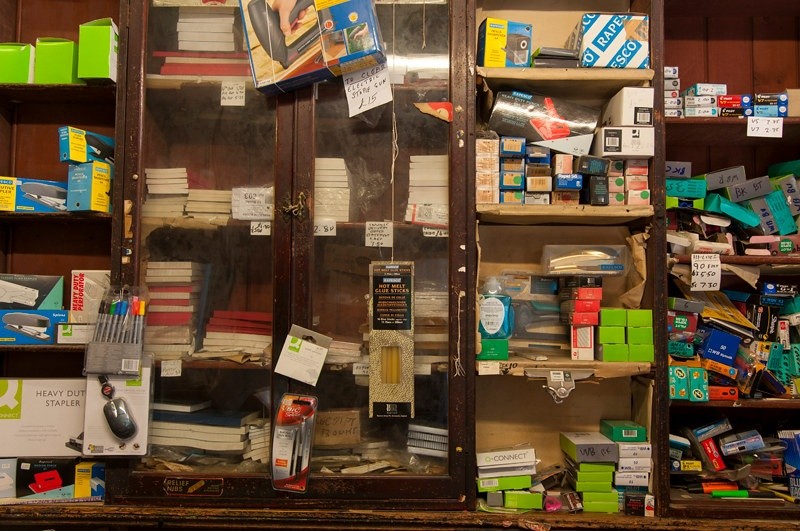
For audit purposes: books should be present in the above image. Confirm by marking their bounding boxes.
[146,399,270,466]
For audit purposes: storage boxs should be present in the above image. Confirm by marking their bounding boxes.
[476,419,654,516]
[478,242,654,363]
[563,11,650,69]
[0,269,112,345]
[239,2,387,95]
[0,16,119,85]
[664,161,798,261]
[0,374,107,508]
[662,285,800,407]
[475,86,654,205]
[667,421,799,507]
[477,17,534,69]
[1,124,113,217]
[664,67,799,121]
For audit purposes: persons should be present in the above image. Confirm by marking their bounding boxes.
[272,0,305,37]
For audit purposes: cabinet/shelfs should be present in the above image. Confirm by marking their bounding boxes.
[475,1,660,529]
[130,1,471,516]
[0,0,124,528]
[666,0,800,530]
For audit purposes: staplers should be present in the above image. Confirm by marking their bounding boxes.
[85,133,113,162]
[2,313,51,340]
[20,182,68,210]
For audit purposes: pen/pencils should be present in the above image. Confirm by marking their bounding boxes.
[94,296,144,343]
[296,425,309,472]
[712,489,776,498]
[759,485,800,503]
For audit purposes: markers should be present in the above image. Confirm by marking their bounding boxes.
[688,482,744,495]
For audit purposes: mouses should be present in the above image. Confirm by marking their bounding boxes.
[103,397,137,440]
